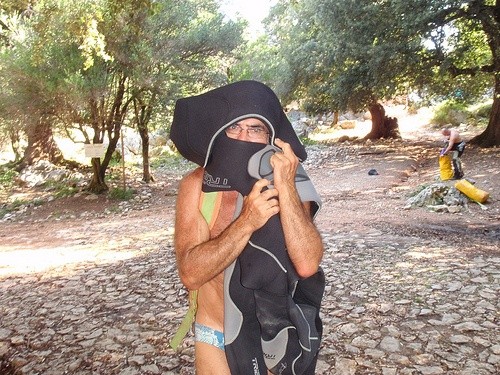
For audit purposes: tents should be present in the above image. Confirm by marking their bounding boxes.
[454,178,490,205]
[438,153,455,181]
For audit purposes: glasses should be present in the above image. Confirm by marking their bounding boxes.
[227,124,271,139]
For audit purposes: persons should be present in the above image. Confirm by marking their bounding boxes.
[169,78,325,375]
[440,127,467,181]
